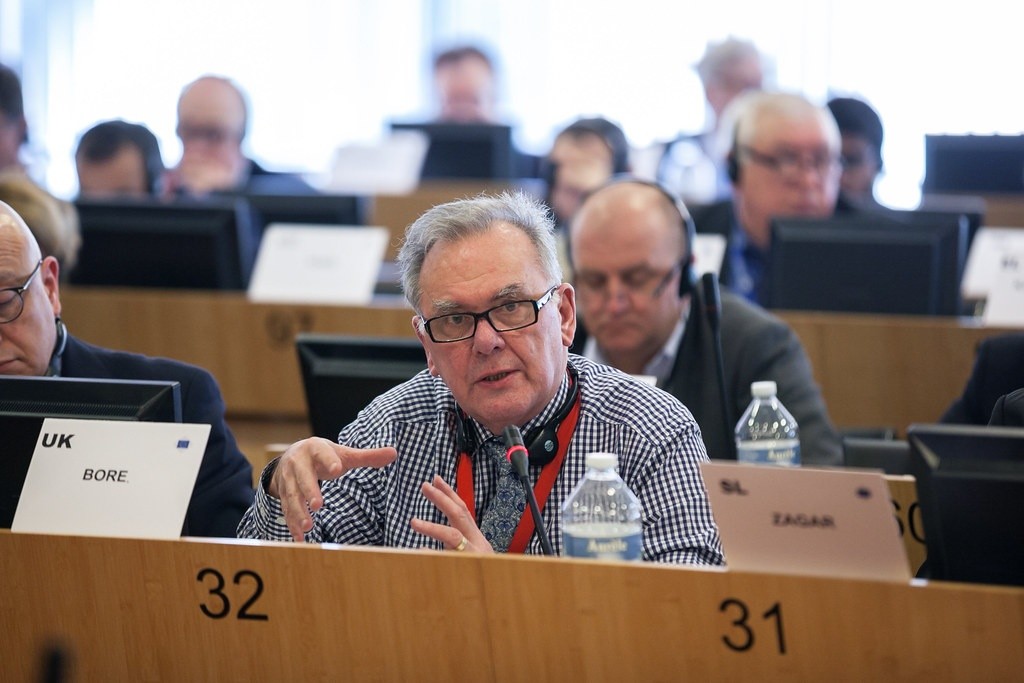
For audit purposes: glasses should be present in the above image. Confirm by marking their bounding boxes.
[735,143,840,175]
[419,284,559,343]
[0,260,43,324]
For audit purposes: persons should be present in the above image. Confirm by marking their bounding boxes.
[655,33,887,307]
[0,198,252,537]
[233,189,724,568]
[171,75,318,287]
[75,119,179,206]
[0,61,81,278]
[937,336,1024,428]
[430,44,543,179]
[558,182,844,469]
[547,117,631,287]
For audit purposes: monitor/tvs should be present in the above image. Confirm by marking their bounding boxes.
[0,118,1024,592]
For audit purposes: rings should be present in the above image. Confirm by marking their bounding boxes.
[455,535,469,550]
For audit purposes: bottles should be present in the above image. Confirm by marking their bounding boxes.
[558,448,645,562]
[734,380,802,468]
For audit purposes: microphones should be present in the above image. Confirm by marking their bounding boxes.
[503,424,554,555]
[702,271,736,466]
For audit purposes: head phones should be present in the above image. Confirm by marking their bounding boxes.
[723,124,745,184]
[567,177,698,297]
[455,360,579,464]
[45,322,67,380]
